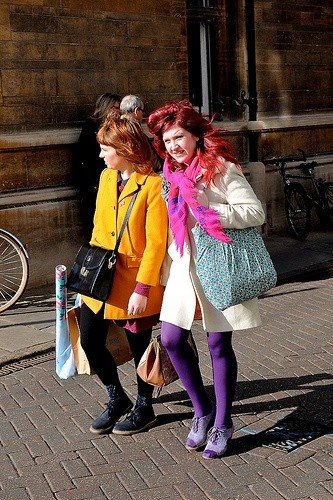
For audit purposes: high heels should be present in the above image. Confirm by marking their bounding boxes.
[201,418,235,459]
[186,405,216,450]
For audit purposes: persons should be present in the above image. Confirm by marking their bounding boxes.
[75,109,169,436]
[77,92,144,242]
[147,99,266,460]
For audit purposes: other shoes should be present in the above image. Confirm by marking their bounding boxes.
[89,393,135,434]
[111,404,158,434]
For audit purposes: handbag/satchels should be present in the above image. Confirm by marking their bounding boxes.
[66,241,119,301]
[74,151,94,181]
[191,220,278,310]
[64,304,135,375]
[137,329,200,388]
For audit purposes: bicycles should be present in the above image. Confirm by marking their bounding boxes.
[263,148,333,240]
[0,229,30,312]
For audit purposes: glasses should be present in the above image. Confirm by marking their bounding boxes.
[133,105,147,115]
